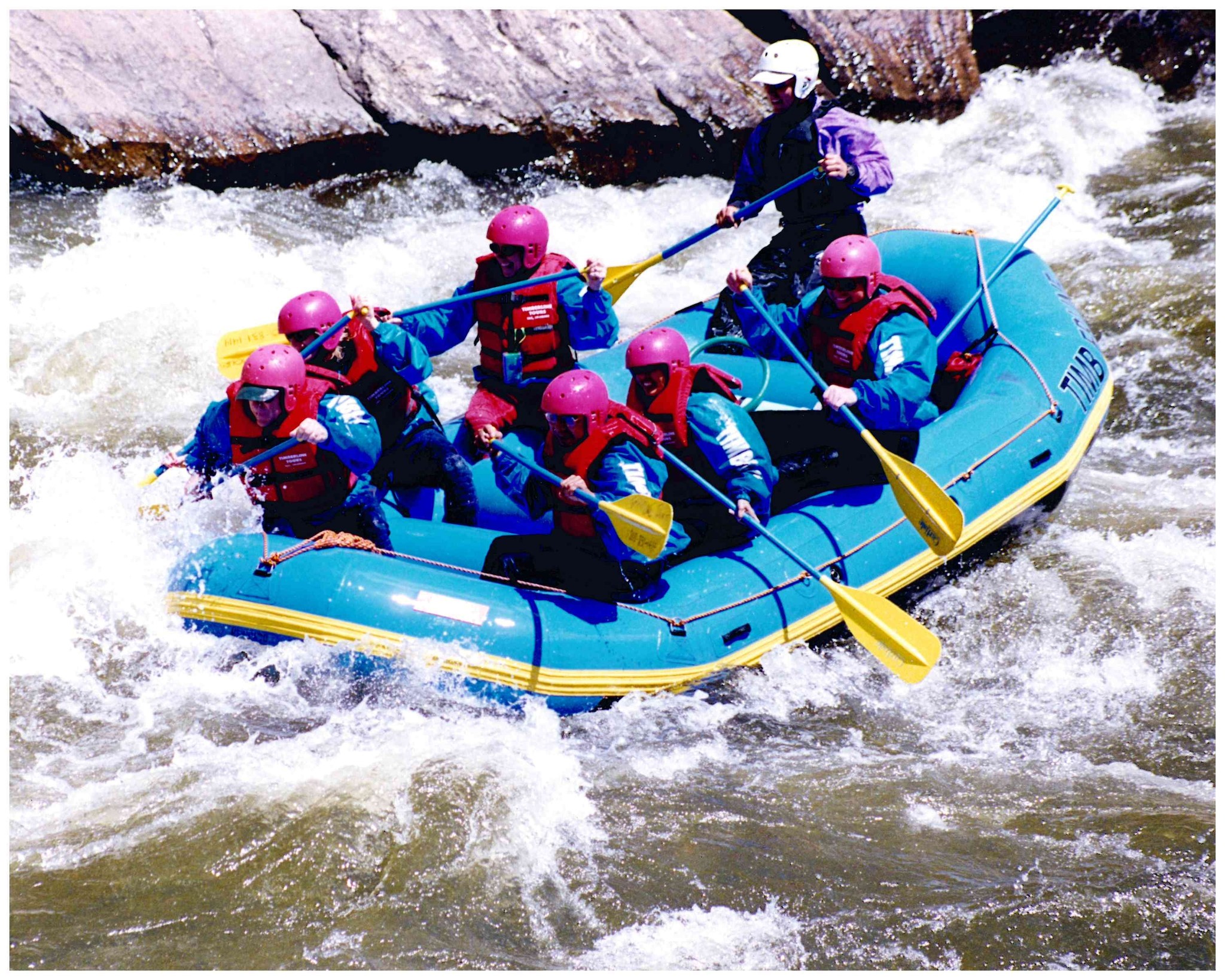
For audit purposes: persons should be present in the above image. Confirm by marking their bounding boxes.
[162,292,479,527]
[473,370,669,602]
[727,235,938,518]
[182,343,397,560]
[702,39,894,358]
[625,326,775,571]
[380,204,620,467]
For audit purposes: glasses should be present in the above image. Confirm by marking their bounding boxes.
[545,412,585,428]
[490,243,523,257]
[763,81,786,90]
[822,276,860,292]
[285,328,317,342]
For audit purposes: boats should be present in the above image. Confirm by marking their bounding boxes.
[166,227,1112,723]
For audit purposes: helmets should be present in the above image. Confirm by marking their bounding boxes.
[277,291,345,350]
[486,205,549,268]
[750,39,822,99]
[233,344,306,424]
[625,328,689,380]
[820,235,880,299]
[541,370,610,431]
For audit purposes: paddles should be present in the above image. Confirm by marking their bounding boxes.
[486,436,674,560]
[656,442,942,687]
[739,279,969,559]
[933,184,1075,350]
[132,435,305,524]
[138,307,369,489]
[580,164,825,307]
[219,264,590,380]
